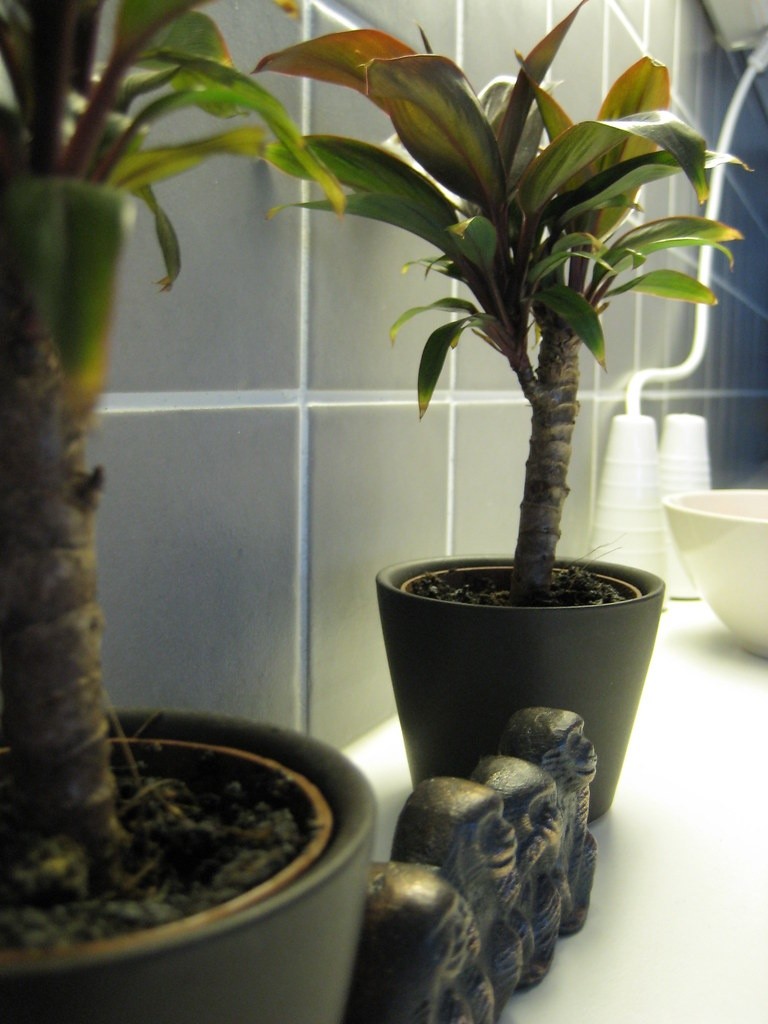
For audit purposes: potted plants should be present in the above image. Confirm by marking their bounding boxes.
[1,1,391,1023]
[249,0,758,823]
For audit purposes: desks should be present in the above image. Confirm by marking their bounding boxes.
[337,582,768,1024]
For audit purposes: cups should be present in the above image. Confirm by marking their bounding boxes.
[588,411,713,611]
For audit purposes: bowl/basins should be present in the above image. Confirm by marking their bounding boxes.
[660,488,768,662]
